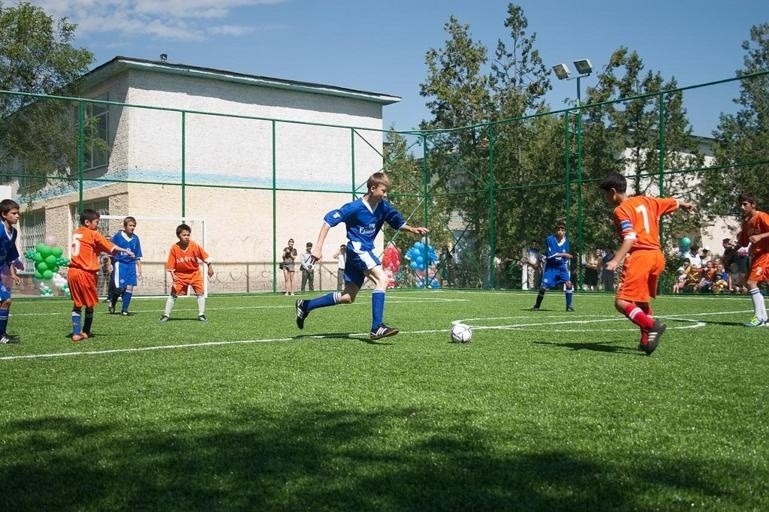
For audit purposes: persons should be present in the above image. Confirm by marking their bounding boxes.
[599,248,618,293]
[278,239,298,296]
[598,172,695,355]
[529,222,576,312]
[158,224,215,323]
[435,246,457,287]
[491,248,506,292]
[583,248,603,293]
[0,199,23,345]
[727,192,769,328]
[332,243,347,292]
[295,171,432,340]
[299,242,316,293]
[523,240,544,290]
[668,238,752,295]
[107,216,143,317]
[99,235,112,298]
[67,208,135,343]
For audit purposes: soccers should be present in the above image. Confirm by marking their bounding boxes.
[451,323,472,342]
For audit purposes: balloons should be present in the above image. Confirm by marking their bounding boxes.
[382,241,441,288]
[24,242,70,296]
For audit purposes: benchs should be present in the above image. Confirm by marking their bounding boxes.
[687,282,696,293]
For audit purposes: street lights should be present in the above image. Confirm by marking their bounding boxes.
[549,59,589,291]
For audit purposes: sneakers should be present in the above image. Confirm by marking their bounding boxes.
[0,334,20,344]
[72,331,94,341]
[745,315,765,327]
[295,299,311,329]
[160,313,168,321]
[122,311,133,315]
[109,301,115,313]
[646,320,666,355]
[370,323,398,341]
[198,315,208,322]
